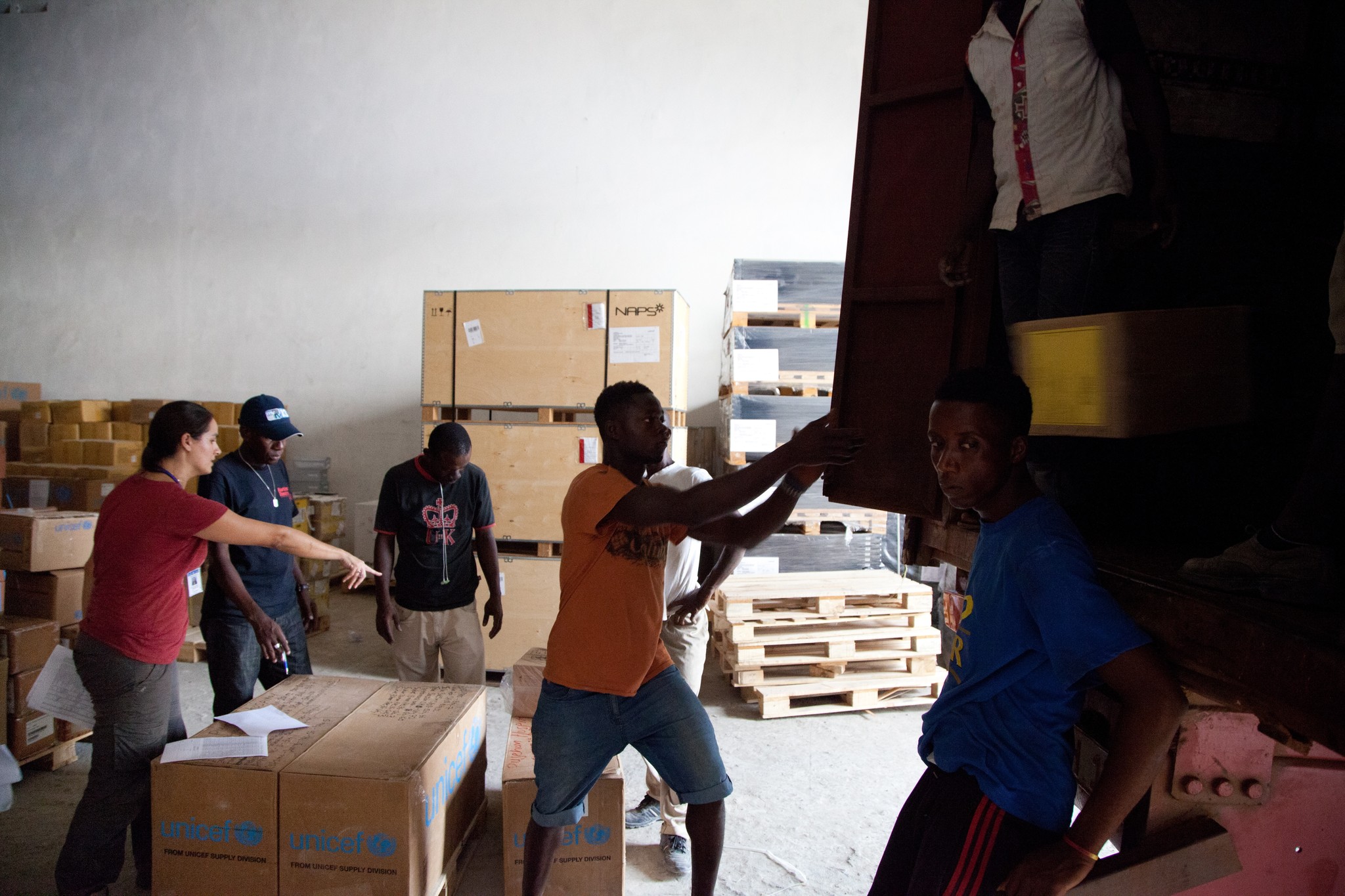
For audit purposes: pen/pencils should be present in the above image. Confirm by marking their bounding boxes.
[283,650,291,676]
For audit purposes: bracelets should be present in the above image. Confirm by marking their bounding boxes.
[295,581,311,594]
[1059,835,1103,863]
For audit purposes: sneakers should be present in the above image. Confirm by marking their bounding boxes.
[625,794,663,829]
[658,833,692,874]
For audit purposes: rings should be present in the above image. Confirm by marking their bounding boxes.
[273,642,281,649]
[358,570,362,574]
[673,612,680,618]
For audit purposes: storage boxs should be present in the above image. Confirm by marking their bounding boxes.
[151,674,391,894]
[512,646,548,719]
[419,420,603,544]
[278,681,492,896]
[419,290,674,411]
[0,382,344,768]
[501,714,626,896]
[1009,307,1216,438]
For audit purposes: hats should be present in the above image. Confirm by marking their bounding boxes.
[238,394,304,442]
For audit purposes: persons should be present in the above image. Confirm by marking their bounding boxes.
[845,353,1184,896]
[50,403,389,896]
[949,1,1147,353]
[511,379,839,896]
[372,423,505,684]
[617,405,746,880]
[196,391,321,727]
